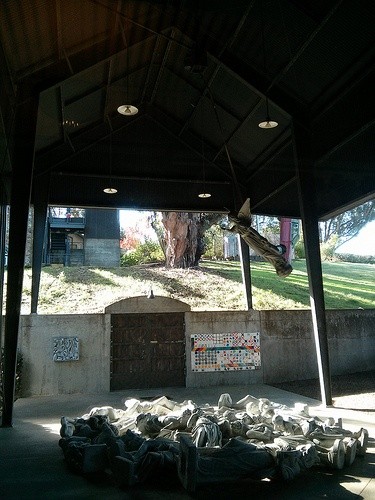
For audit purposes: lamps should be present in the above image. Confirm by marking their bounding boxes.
[197,140,211,198]
[117,69,138,116]
[103,136,117,194]
[258,94,279,129]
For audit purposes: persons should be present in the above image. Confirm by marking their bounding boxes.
[272,415,363,442]
[176,433,320,490]
[58,414,147,480]
[91,394,195,419]
[191,415,223,449]
[218,394,309,426]
[137,408,203,438]
[112,438,176,490]
[232,420,357,465]
[220,197,293,279]
